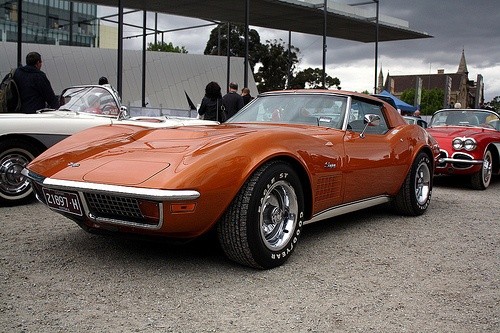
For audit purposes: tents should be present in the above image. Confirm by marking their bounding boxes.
[377,89,419,113]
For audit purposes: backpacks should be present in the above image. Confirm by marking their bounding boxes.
[0,68,22,113]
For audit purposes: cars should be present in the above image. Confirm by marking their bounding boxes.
[425,109,500,189]
[403,117,428,128]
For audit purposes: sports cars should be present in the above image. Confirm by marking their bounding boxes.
[0,86,221,205]
[21,90,440,267]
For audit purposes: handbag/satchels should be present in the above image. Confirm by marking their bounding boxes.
[220,111,228,123]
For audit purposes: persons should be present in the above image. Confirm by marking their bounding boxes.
[241,87,255,106]
[98,76,122,107]
[198,81,223,123]
[84,94,103,114]
[223,82,244,122]
[0,52,60,114]
[446,102,461,127]
